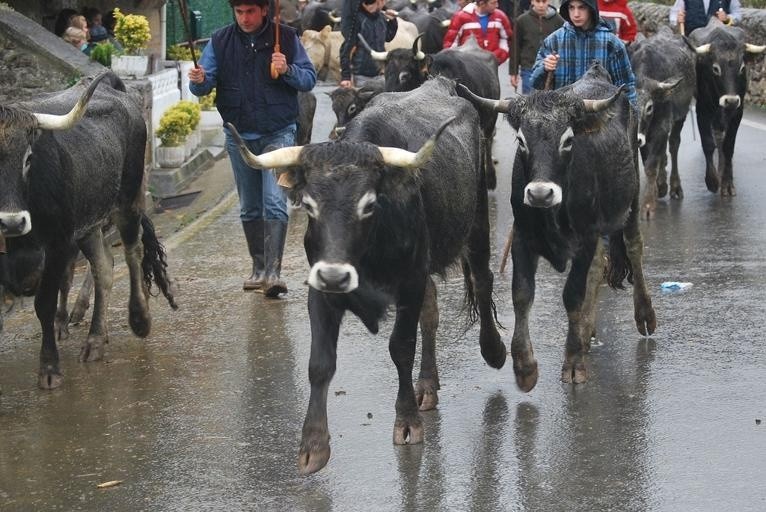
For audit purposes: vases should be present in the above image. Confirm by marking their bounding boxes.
[107,5,151,79]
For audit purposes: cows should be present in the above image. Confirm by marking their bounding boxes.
[458,59,656,392]
[0,217,117,340]
[357,32,501,190]
[323,81,387,139]
[625,26,697,221]
[224,72,507,477]
[679,15,766,197]
[0,71,178,390]
[278,0,459,86]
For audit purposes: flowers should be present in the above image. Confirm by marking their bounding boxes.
[151,86,221,167]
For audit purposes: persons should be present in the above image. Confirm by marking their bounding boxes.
[56,8,124,65]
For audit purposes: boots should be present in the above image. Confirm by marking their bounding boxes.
[242,220,264,290]
[262,218,289,297]
